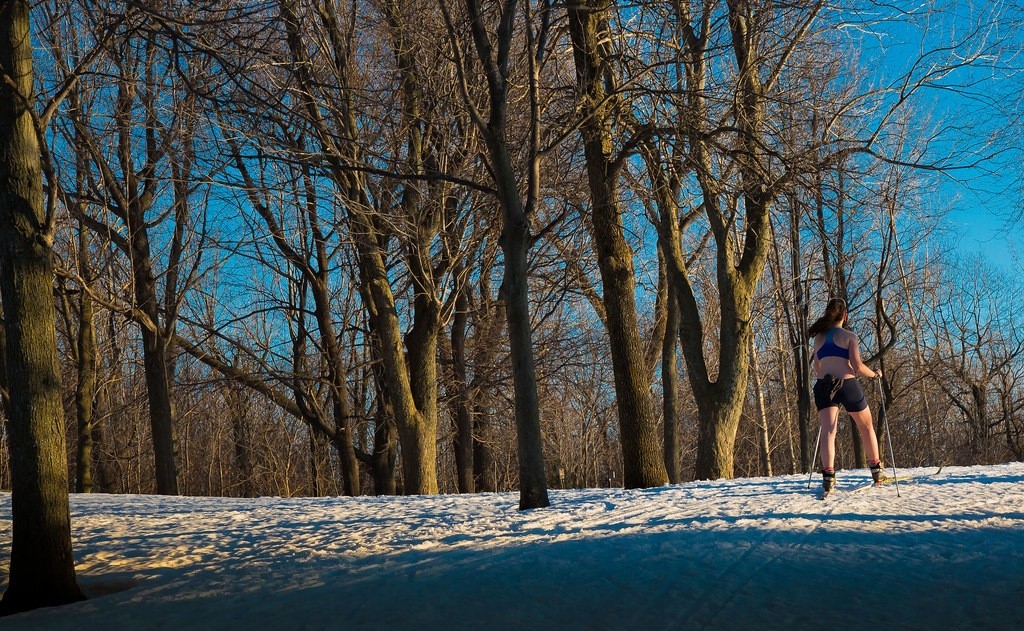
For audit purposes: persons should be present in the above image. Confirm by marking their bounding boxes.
[805,300,883,498]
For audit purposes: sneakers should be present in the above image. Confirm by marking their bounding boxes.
[820,469,836,491]
[866,460,887,483]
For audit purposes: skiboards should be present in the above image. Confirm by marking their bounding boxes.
[819,465,942,501]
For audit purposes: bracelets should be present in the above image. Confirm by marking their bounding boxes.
[873,373,879,379]
